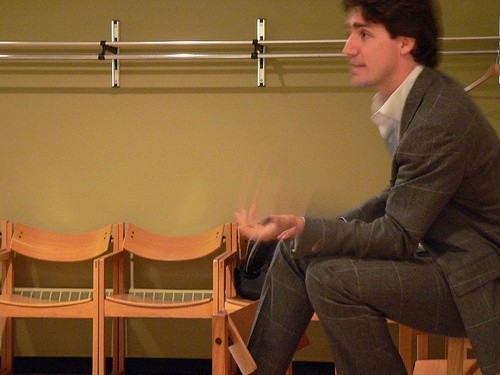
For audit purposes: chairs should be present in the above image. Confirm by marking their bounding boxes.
[0,218,498,375]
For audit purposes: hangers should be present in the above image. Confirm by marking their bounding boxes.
[463,53,499,94]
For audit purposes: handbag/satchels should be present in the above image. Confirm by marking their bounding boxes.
[234,223,276,300]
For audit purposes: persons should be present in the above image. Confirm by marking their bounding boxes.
[234,0,500,375]
